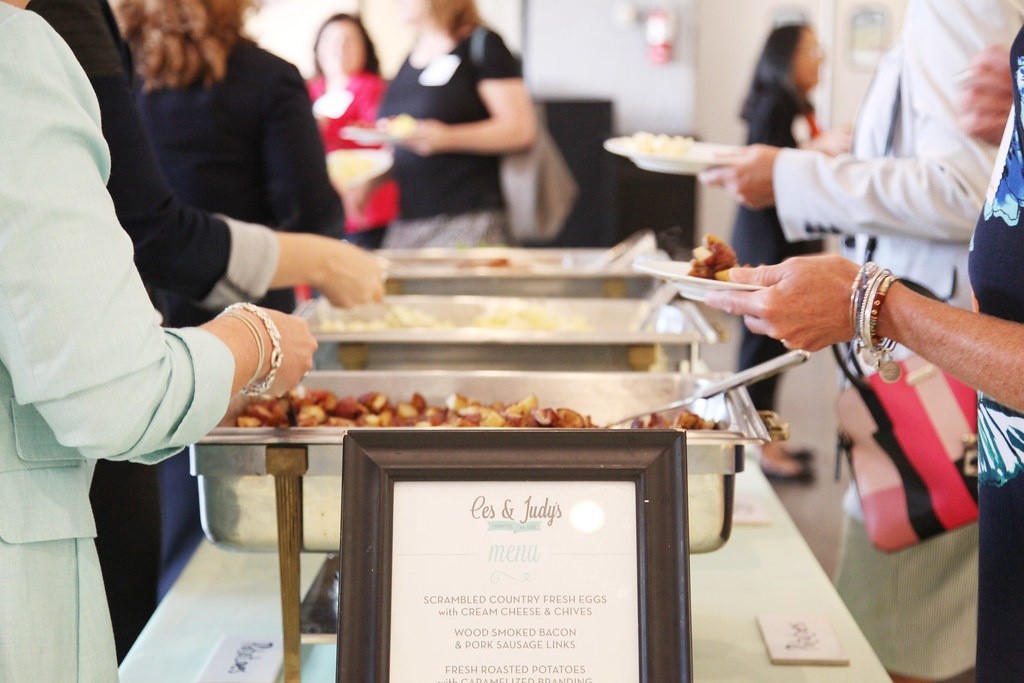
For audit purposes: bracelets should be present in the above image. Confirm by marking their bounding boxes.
[223,302,284,396]
[849,261,903,381]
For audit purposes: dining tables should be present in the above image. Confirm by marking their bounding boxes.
[109,365,895,680]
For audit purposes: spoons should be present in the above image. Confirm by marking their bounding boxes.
[591,229,657,274]
[630,280,680,332]
[601,346,808,431]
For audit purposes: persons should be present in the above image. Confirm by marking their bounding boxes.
[706,21,1024,683]
[727,23,828,484]
[623,10,699,138]
[0,0,318,683]
[379,0,542,247]
[105,0,347,612]
[303,15,396,252]
[698,2,1024,682]
[0,2,388,662]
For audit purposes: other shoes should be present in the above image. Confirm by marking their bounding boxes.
[761,450,816,488]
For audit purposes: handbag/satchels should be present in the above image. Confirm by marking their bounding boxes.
[838,347,982,554]
[496,105,578,238]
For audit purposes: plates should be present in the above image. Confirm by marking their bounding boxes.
[339,125,424,146]
[327,150,395,184]
[631,258,771,302]
[603,136,745,176]
